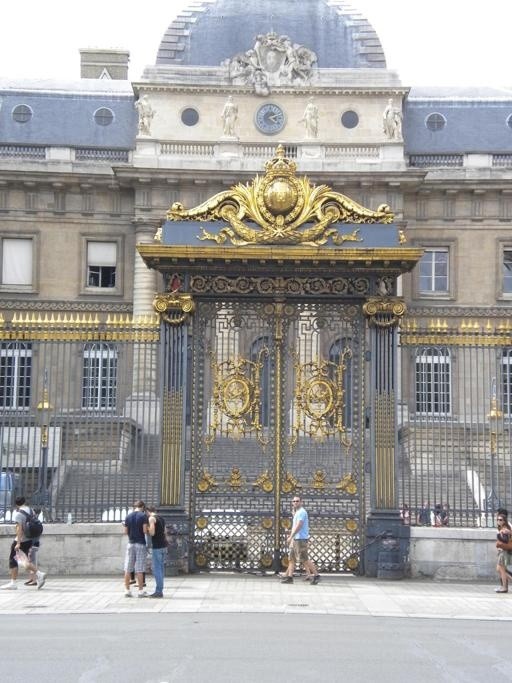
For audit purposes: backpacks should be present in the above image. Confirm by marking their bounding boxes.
[16,506,43,538]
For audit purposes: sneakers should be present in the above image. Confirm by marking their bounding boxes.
[124,590,163,598]
[0,581,19,590]
[303,573,320,586]
[24,570,47,590]
[277,571,294,584]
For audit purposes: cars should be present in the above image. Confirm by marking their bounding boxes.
[100,505,140,522]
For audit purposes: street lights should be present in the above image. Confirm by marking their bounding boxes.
[37,386,55,520]
[485,392,504,514]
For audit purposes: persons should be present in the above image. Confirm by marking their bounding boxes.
[122,499,148,597]
[0,495,48,590]
[282,496,321,585]
[302,95,318,139]
[139,94,155,135]
[383,98,402,139]
[23,506,40,586]
[125,506,151,587]
[399,501,451,527]
[146,504,169,597]
[280,504,314,581]
[222,94,237,134]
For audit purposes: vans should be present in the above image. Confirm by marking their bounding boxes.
[195,508,252,562]
[1,472,25,521]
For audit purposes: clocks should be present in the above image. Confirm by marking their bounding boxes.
[255,101,287,133]
